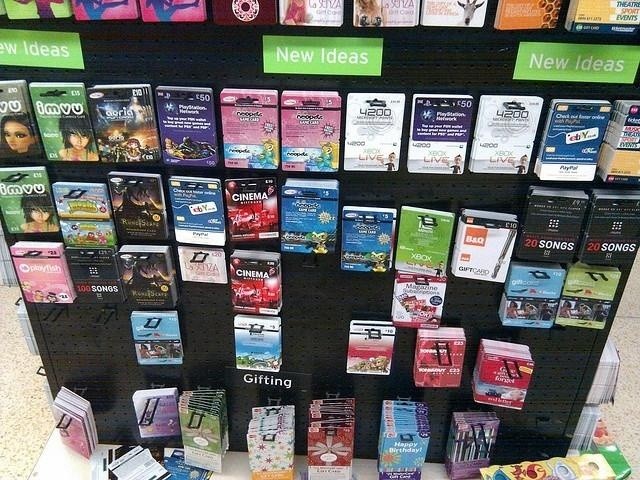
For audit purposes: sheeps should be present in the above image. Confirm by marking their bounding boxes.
[457,0,485,26]
